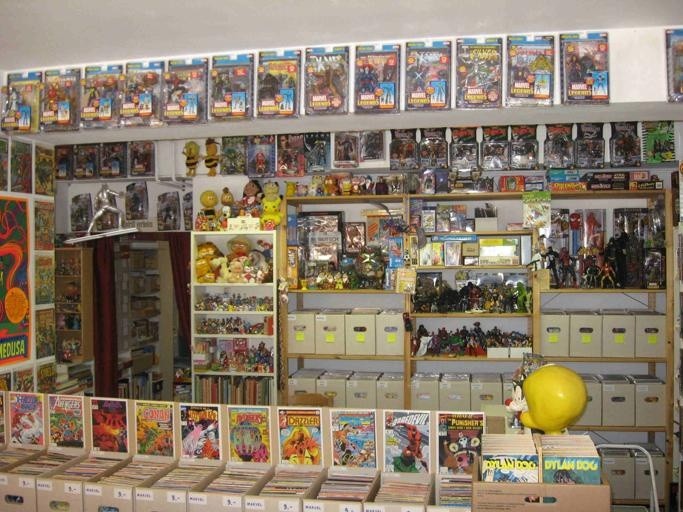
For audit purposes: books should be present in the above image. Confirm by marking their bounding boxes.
[109,372,272,406]
[1,391,610,510]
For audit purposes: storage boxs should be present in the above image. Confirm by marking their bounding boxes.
[345,371,382,407]
[289,309,323,354]
[511,347,532,358]
[488,347,510,358]
[0,99,683,512]
[316,369,352,408]
[409,371,440,410]
[630,311,664,357]
[376,372,404,408]
[498,369,515,406]
[630,441,663,501]
[344,308,381,356]
[566,374,601,427]
[565,311,603,358]
[598,311,636,359]
[543,312,569,356]
[314,310,349,355]
[597,374,635,427]
[470,372,503,413]
[598,445,636,501]
[288,367,324,395]
[376,309,404,356]
[628,372,665,427]
[438,371,471,411]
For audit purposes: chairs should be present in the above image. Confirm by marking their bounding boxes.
[283,390,336,408]
